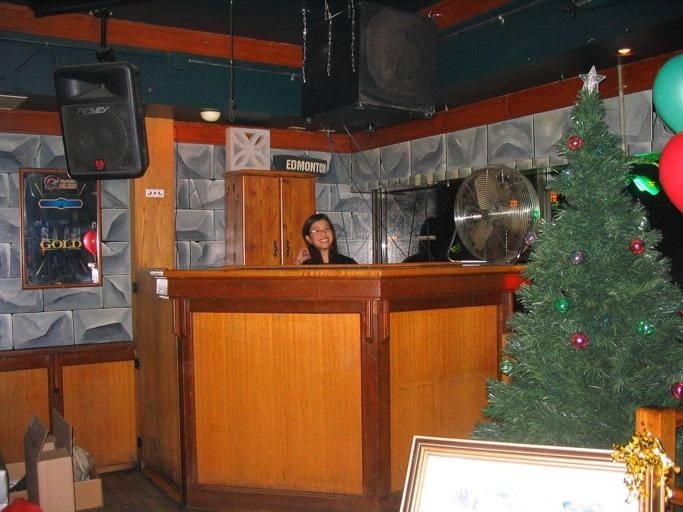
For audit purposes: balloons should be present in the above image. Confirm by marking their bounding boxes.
[658,131,683,213]
[652,54,683,134]
[82,231,97,257]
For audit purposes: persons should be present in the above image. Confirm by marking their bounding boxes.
[300,213,359,265]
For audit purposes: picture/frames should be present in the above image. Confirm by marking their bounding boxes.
[398,434,667,512]
[17,165,104,291]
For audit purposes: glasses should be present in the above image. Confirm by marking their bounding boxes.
[306,226,333,236]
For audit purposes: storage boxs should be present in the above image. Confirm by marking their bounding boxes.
[4,408,106,511]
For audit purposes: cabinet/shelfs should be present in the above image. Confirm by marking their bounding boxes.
[222,169,317,269]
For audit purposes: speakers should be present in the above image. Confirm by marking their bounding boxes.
[53,62,150,181]
[300,1,439,134]
